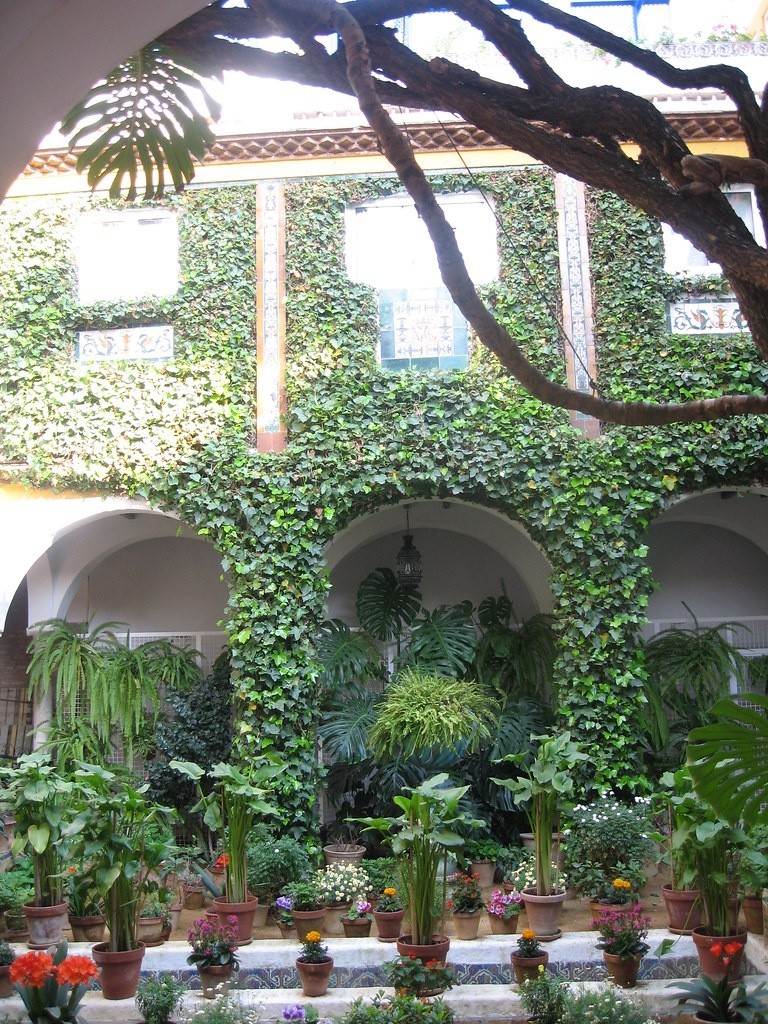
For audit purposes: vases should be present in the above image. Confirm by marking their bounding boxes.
[366,896,382,913]
[488,913,519,934]
[520,833,567,871]
[340,912,372,937]
[373,907,405,937]
[452,908,484,940]
[296,955,334,997]
[277,920,298,939]
[199,962,234,998]
[252,904,271,927]
[510,949,548,988]
[589,900,633,928]
[693,1010,746,1024]
[603,950,641,988]
[211,867,227,892]
[321,901,353,934]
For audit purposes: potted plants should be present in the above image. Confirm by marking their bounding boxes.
[489,731,592,935]
[280,881,327,943]
[465,838,503,887]
[168,760,281,940]
[644,792,703,928]
[342,772,487,987]
[323,812,367,868]
[497,843,536,910]
[0,751,207,1000]
[659,757,747,980]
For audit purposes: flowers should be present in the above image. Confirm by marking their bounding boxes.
[216,850,232,868]
[485,890,522,919]
[509,964,662,1024]
[598,879,633,907]
[664,940,768,1024]
[590,904,655,961]
[338,950,462,1024]
[517,927,541,958]
[134,970,270,1024]
[186,912,242,970]
[275,857,400,916]
[297,929,331,964]
[512,856,568,894]
[560,791,653,839]
[8,936,102,1024]
[274,1002,336,1024]
[446,871,486,913]
